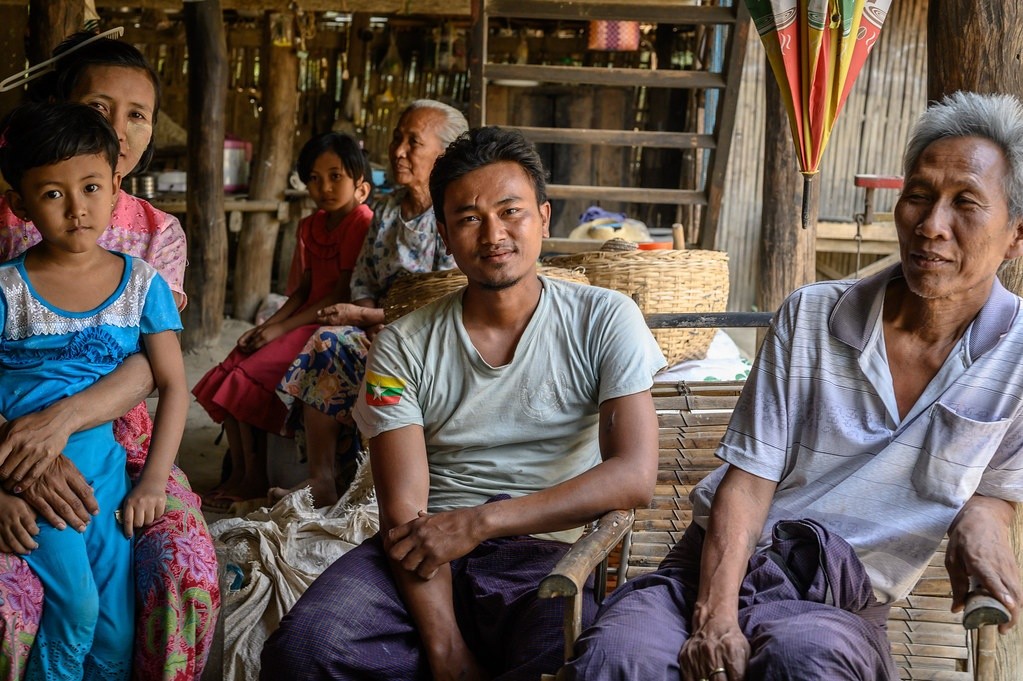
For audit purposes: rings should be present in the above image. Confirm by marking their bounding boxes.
[707,667,726,677]
[699,678,710,681]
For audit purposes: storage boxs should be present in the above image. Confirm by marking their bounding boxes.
[223,140,252,192]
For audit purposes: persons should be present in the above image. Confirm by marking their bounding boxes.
[225,100,469,508]
[353,157,374,209]
[198,130,377,512]
[1,96,190,681]
[259,121,658,681]
[0,33,225,681]
[553,88,1022,681]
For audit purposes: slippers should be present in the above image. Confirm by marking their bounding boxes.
[201,476,277,511]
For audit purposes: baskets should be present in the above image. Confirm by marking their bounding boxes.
[541,249,729,373]
[383,264,589,323]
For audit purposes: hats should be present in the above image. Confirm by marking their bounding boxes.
[567,217,654,242]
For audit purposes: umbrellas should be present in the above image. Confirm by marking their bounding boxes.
[745,0,893,230]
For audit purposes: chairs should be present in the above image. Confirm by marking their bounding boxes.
[534,307,1023,681]
